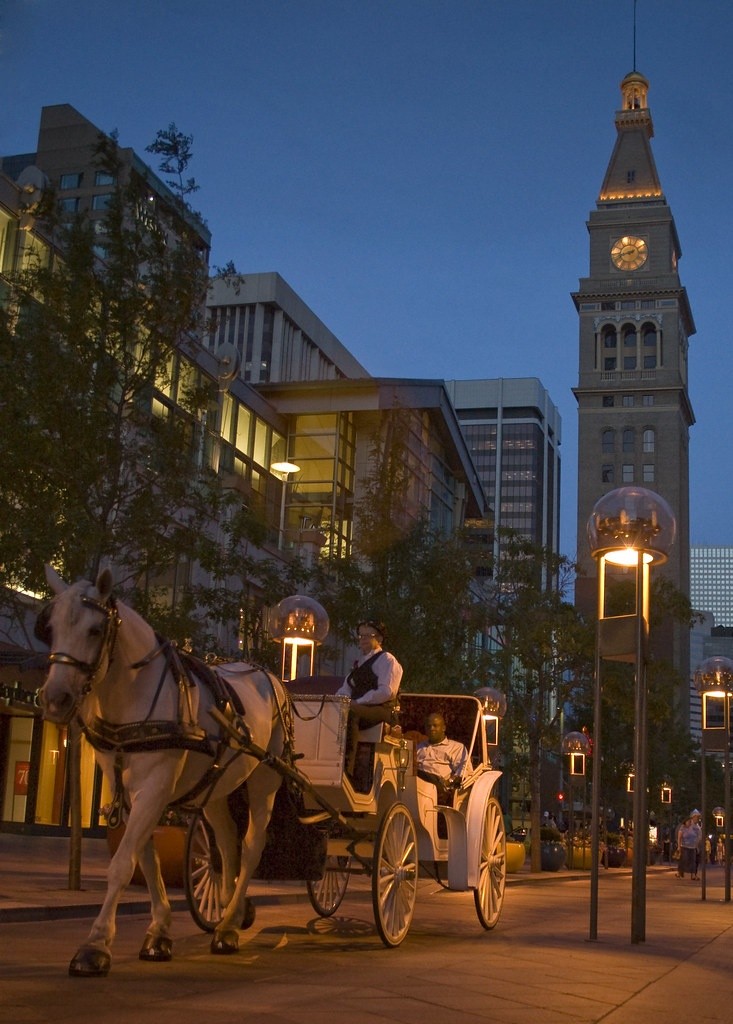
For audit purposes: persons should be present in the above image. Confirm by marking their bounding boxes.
[501,808,513,836]
[677,808,703,880]
[616,826,634,837]
[600,821,604,837]
[416,712,473,836]
[706,836,725,866]
[662,835,670,864]
[543,812,568,836]
[335,617,404,791]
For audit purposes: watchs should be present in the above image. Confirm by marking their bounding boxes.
[449,778,454,783]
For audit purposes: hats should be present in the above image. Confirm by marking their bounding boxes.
[356,618,386,637]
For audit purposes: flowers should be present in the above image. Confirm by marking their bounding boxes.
[561,829,607,852]
[97,795,203,827]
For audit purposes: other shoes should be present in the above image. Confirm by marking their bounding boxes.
[691,875,700,880]
[675,873,682,880]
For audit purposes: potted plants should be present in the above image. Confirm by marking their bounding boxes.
[598,832,625,868]
[537,826,562,868]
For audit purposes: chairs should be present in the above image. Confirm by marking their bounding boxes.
[283,675,394,796]
[398,693,491,839]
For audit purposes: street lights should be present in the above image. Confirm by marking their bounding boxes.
[267,594,329,683]
[694,657,733,903]
[712,807,724,835]
[623,759,633,871]
[657,774,676,864]
[558,732,588,871]
[474,687,506,768]
[584,486,676,950]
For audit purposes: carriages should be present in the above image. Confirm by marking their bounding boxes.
[34,554,508,979]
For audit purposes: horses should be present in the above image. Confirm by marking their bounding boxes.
[38,562,295,979]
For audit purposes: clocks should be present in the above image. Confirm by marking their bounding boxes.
[611,236,648,271]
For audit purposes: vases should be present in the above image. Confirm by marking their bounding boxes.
[562,845,602,870]
[506,838,524,872]
[106,824,185,890]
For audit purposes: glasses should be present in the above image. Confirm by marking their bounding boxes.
[359,633,376,639]
